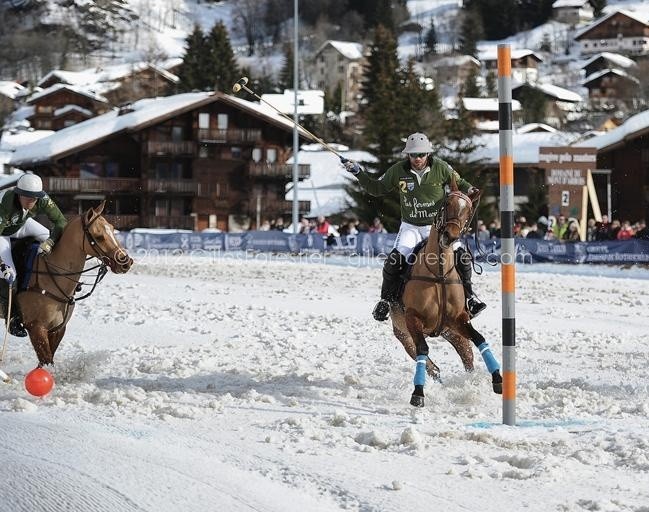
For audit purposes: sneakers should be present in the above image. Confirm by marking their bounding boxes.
[469,298,486,315]
[374,300,392,317]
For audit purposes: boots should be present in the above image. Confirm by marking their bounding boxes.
[2,303,27,337]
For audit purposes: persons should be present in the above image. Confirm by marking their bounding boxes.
[259,215,387,234]
[0,172,68,337]
[340,131,487,321]
[461,213,649,241]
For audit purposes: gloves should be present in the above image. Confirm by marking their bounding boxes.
[1,263,15,282]
[468,187,480,198]
[37,238,54,255]
[341,158,360,175]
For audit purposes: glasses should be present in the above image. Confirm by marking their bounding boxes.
[409,153,427,158]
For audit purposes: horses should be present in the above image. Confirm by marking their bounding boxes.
[0,200,133,368]
[389,171,503,408]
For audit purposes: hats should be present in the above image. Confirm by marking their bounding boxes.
[12,172,46,198]
[402,132,436,154]
